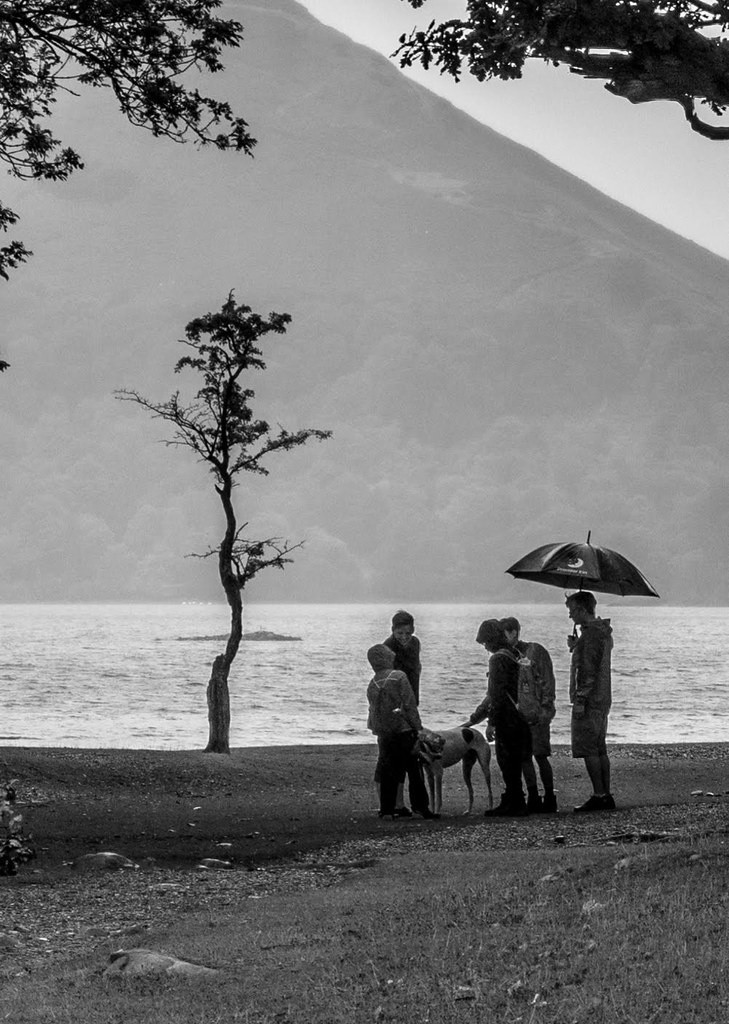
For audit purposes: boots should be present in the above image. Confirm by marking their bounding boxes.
[485,792,526,816]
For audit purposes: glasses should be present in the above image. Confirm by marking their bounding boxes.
[569,608,577,615]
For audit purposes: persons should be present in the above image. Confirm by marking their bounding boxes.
[367,610,439,818]
[457,613,557,817]
[565,591,615,812]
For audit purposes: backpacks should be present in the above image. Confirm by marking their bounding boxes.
[370,670,405,737]
[494,646,543,726]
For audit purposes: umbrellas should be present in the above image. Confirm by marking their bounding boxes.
[504,530,660,652]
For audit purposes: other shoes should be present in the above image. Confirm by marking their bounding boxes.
[411,806,440,819]
[542,795,557,812]
[395,808,412,817]
[527,796,544,812]
[573,794,616,812]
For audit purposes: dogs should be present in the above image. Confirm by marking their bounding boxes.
[417,725,494,815]
